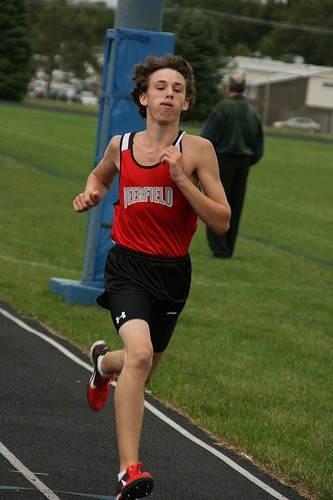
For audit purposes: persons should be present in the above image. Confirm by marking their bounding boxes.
[72,55,231,500]
[200,75,264,259]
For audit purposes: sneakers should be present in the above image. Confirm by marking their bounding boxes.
[87,340,115,412]
[115,461,154,500]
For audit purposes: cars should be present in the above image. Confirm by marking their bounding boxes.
[73,90,97,105]
[273,116,321,132]
